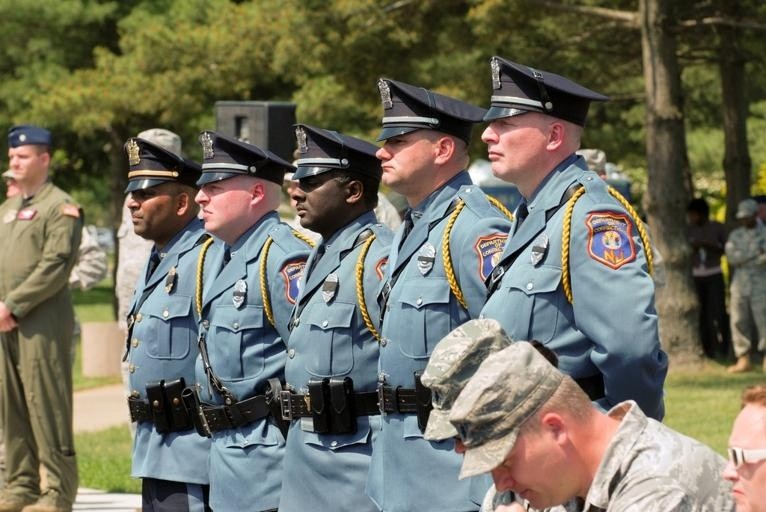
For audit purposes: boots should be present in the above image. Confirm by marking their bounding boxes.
[728,353,753,373]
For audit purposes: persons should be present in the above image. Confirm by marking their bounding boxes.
[446,337,737,512]
[282,159,405,247]
[718,382,766,512]
[575,149,607,182]
[271,117,398,511]
[62,224,109,294]
[477,52,670,426]
[0,122,87,512]
[121,133,229,512]
[684,196,732,359]
[753,194,766,227]
[724,198,766,376]
[373,75,515,512]
[3,168,21,199]
[191,128,321,512]
[112,127,185,439]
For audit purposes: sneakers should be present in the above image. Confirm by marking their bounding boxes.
[22,491,71,512]
[0,492,26,512]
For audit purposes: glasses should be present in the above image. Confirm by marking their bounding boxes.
[724,445,766,473]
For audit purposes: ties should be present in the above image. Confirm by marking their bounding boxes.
[307,243,325,280]
[221,248,230,273]
[144,251,158,284]
[396,207,414,250]
[514,201,528,234]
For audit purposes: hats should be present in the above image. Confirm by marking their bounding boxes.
[483,55,611,128]
[7,125,53,148]
[123,136,200,193]
[290,121,383,182]
[447,340,566,483]
[137,125,183,158]
[736,200,759,220]
[195,129,298,186]
[576,148,606,173]
[374,76,489,143]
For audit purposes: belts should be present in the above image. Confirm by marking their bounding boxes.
[566,375,607,402]
[193,393,270,439]
[126,396,196,424]
[281,390,382,419]
[376,387,419,417]
